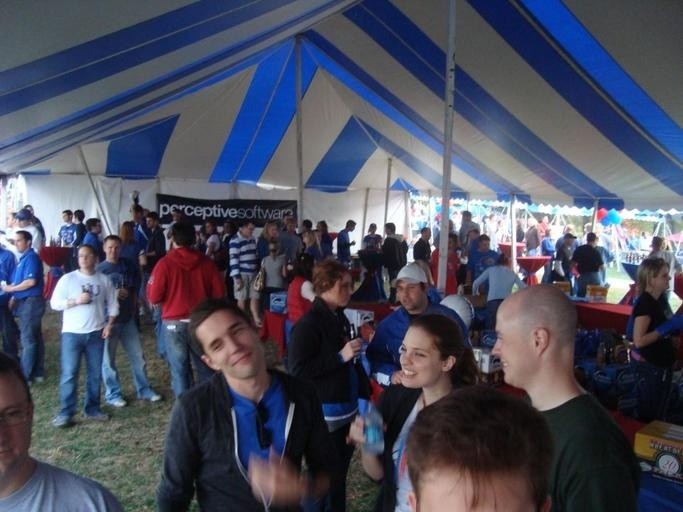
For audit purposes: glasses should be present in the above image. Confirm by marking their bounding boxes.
[256,403,272,449]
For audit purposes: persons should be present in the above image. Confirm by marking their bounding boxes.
[156,253,681,510]
[0,351,123,511]
[0,205,46,386]
[51,202,357,429]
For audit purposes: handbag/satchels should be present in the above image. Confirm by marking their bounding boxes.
[253,269,265,292]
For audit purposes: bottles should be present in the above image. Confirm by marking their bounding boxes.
[361,401,385,456]
[347,324,361,364]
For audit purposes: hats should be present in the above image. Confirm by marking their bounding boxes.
[13,209,32,219]
[390,263,427,287]
[564,234,577,239]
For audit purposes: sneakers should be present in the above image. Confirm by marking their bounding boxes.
[52,392,162,426]
